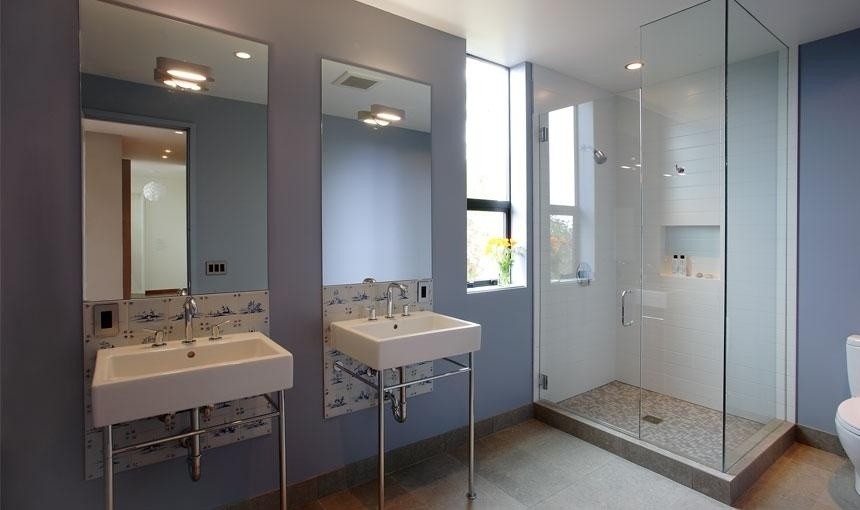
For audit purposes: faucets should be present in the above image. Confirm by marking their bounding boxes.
[363,278,376,285]
[386,281,408,318]
[183,298,197,342]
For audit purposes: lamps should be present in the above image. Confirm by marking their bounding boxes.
[358,104,406,127]
[153,56,212,91]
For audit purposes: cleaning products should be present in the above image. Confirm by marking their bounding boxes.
[672,254,677,276]
[678,255,686,276]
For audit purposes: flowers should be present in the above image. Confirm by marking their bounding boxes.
[483,234,525,285]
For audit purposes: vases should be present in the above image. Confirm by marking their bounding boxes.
[498,264,510,287]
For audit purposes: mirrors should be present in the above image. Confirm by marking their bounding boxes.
[320,56,432,286]
[79,0,268,303]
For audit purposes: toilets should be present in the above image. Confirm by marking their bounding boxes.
[836,334,860,488]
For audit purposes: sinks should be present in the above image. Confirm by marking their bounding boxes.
[634,286,684,310]
[91,330,294,427]
[330,310,482,371]
